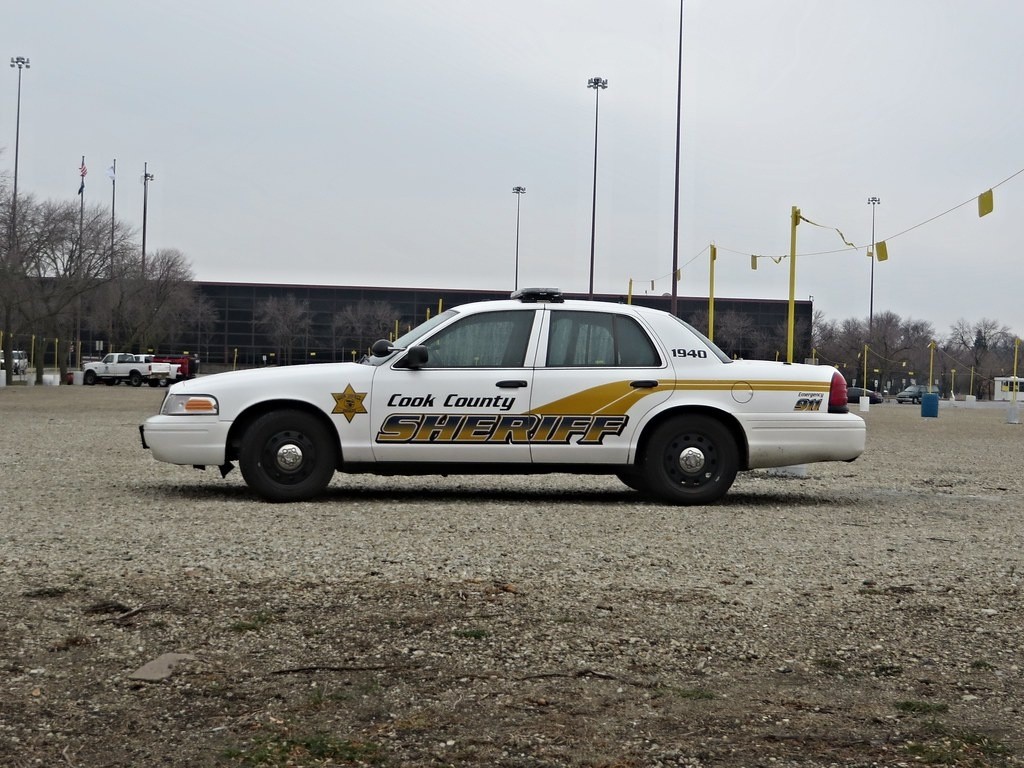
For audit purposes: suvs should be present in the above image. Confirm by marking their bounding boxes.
[0,348,28,376]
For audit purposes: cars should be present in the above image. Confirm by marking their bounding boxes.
[896,385,941,404]
[844,387,884,405]
[139,285,867,506]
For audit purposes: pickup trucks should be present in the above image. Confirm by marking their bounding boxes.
[112,354,185,386]
[82,352,171,387]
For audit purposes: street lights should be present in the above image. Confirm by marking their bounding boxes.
[587,77,608,302]
[864,196,881,388]
[5,56,31,384]
[513,186,527,292]
